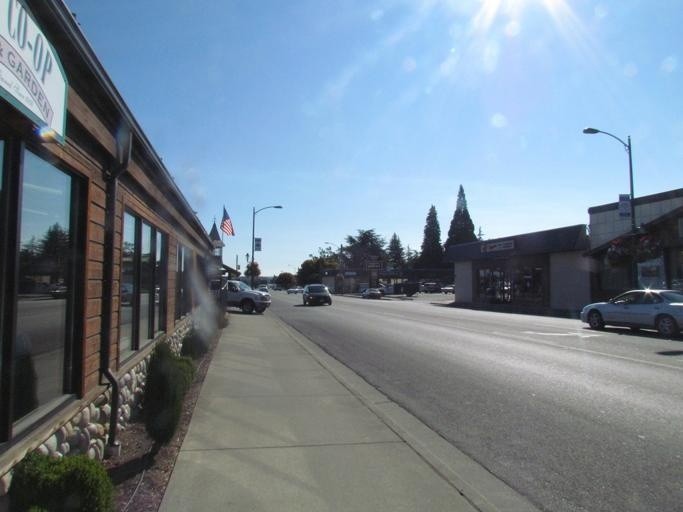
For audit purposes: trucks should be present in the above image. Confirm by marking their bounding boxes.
[376,272,419,296]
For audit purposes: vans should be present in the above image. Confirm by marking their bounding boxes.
[424,283,440,293]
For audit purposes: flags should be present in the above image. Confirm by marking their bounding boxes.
[219,208,235,236]
[208,223,225,248]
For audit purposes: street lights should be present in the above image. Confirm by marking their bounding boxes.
[583,128,639,289]
[251,205,282,290]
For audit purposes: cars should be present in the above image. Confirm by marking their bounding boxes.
[154,287,160,304]
[228,280,272,314]
[258,284,268,292]
[121,282,133,307]
[287,284,332,305]
[51,286,67,299]
[440,285,455,294]
[361,288,384,299]
[580,289,683,339]
